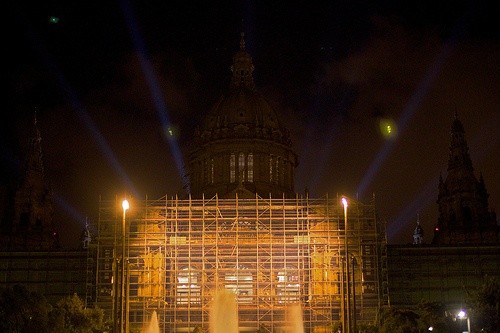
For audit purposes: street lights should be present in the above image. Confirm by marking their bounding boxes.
[457,311,471,333]
[341,197,352,333]
[120,200,130,333]
